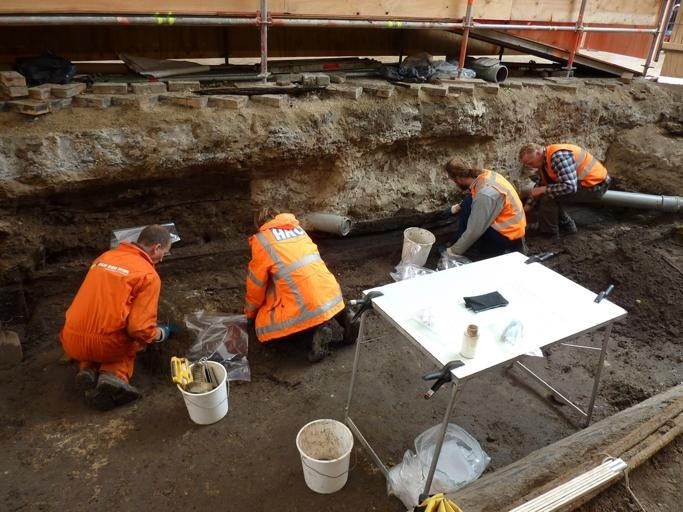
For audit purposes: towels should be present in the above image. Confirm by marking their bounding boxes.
[464,291,507,313]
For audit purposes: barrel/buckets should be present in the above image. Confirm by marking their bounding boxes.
[176,360,231,426]
[295,418,353,494]
[401,227,437,269]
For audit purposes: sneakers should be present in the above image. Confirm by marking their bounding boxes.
[96,373,142,404]
[75,369,115,411]
[307,326,332,362]
[515,238,528,256]
[338,312,360,346]
[528,236,561,249]
[559,219,577,234]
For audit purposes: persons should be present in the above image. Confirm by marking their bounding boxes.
[519,143,611,237]
[59,225,172,411]
[438,157,527,260]
[244,204,362,362]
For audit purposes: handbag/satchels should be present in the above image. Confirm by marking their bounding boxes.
[15,55,73,85]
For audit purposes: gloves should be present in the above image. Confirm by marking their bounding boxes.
[435,208,452,219]
[154,324,171,344]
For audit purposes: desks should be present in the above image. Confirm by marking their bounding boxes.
[342,250,629,512]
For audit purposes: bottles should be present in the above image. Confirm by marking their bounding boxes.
[460,324,480,359]
